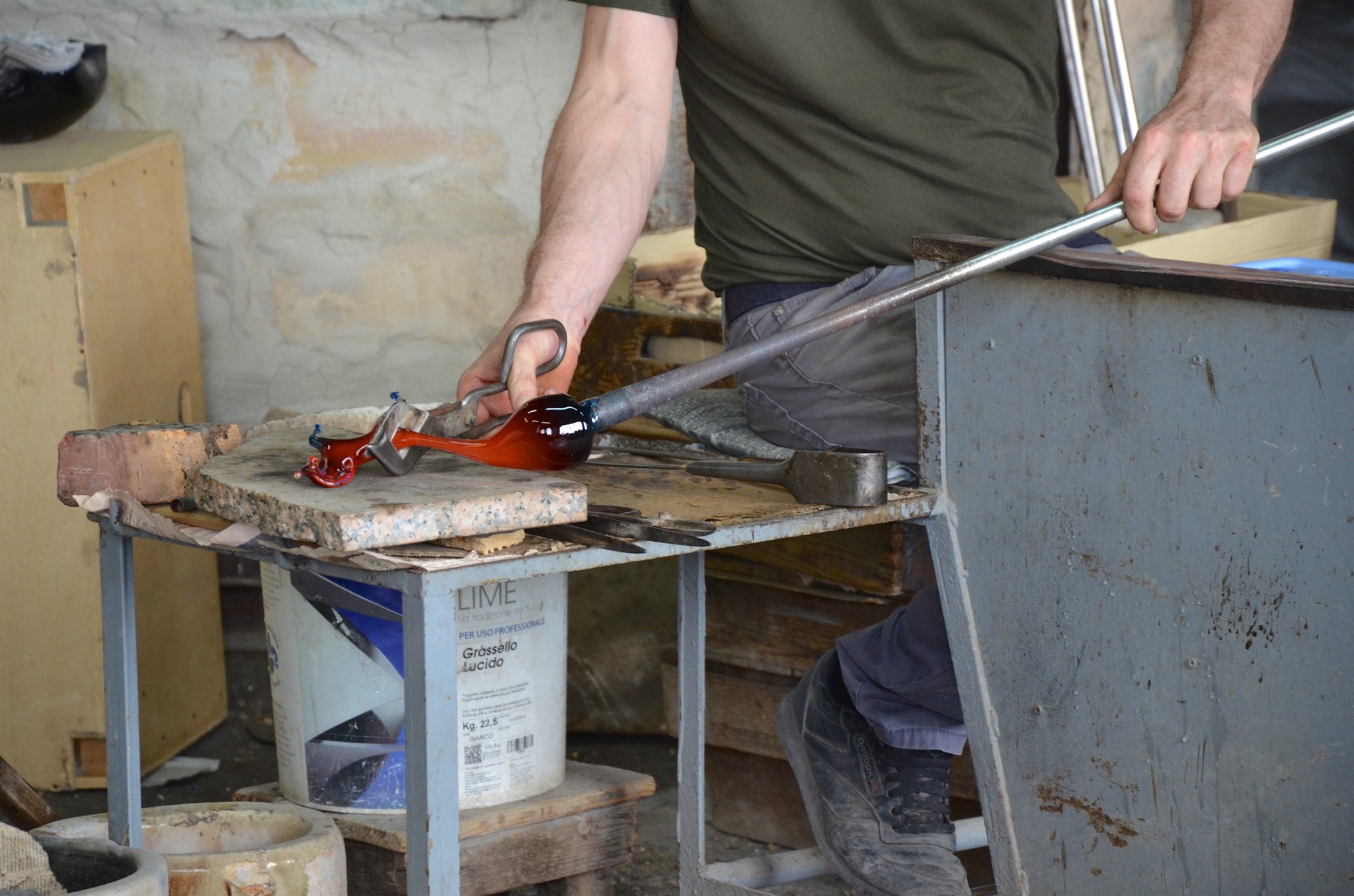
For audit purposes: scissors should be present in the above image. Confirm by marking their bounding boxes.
[586,504,716,537]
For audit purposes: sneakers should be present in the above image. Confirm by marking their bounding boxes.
[777,646,971,896]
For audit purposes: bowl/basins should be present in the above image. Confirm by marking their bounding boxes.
[0,43,108,142]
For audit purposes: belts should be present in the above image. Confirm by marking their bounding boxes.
[722,278,838,328]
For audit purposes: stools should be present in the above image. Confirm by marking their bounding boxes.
[232,759,656,896]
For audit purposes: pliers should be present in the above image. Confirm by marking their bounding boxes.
[366,319,568,476]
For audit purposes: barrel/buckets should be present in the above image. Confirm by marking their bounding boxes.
[258,560,568,818]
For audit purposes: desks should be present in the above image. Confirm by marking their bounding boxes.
[0,127,227,793]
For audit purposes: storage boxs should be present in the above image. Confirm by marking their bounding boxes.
[567,191,1338,800]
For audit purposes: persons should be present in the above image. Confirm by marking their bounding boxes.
[456,0,1300,896]
